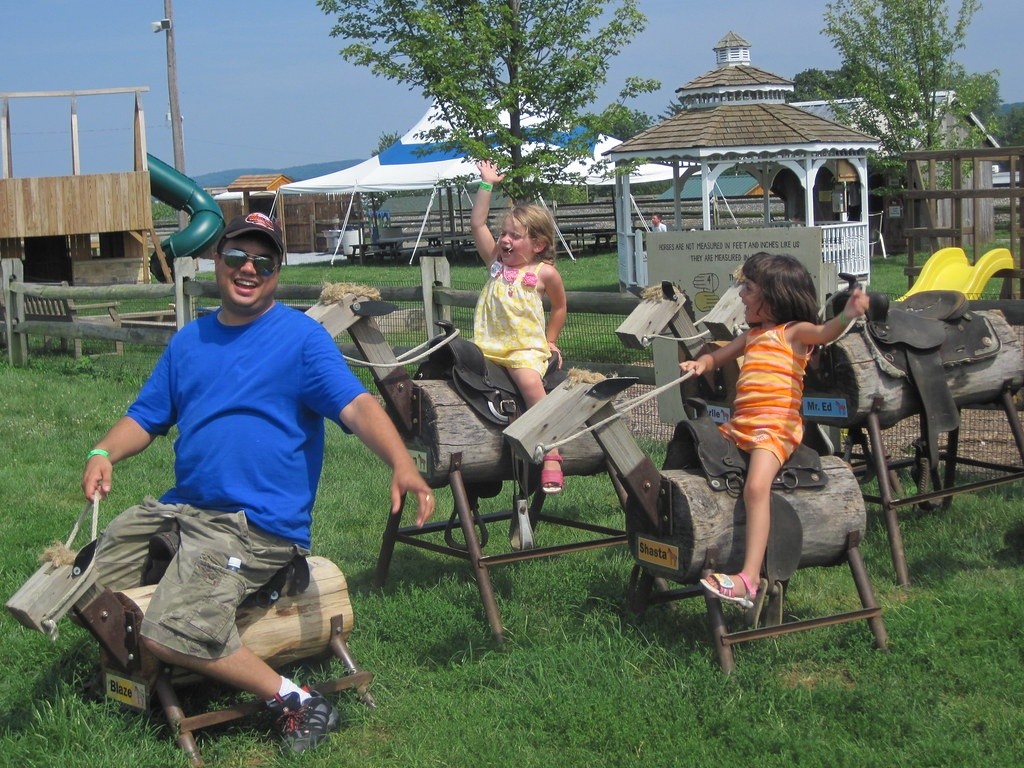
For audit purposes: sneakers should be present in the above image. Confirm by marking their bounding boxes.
[276,690,339,761]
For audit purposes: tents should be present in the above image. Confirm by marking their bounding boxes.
[267,94,711,265]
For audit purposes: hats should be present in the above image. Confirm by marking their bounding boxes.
[216,212,284,258]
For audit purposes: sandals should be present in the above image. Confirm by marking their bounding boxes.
[701,572,759,608]
[542,455,564,493]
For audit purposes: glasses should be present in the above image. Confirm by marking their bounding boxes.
[219,250,279,276]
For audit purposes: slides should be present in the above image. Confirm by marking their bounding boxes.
[144,149,226,284]
[893,244,1017,307]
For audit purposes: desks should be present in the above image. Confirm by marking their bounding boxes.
[437,234,475,264]
[379,234,442,266]
[555,222,595,251]
[349,240,400,263]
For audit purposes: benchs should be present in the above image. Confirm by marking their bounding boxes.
[342,231,618,264]
[22,280,125,361]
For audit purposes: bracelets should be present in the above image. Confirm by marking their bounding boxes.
[87,448,108,460]
[838,312,851,326]
[479,182,492,191]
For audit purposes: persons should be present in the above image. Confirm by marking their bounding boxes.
[679,253,869,611]
[651,212,667,232]
[470,162,567,493]
[82,213,436,759]
